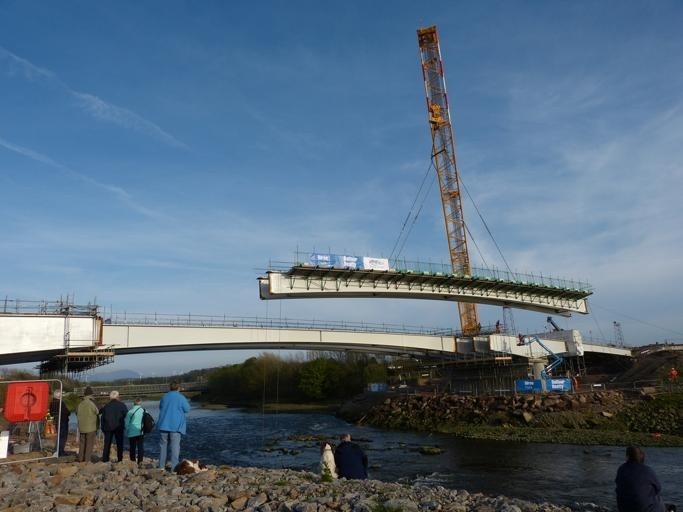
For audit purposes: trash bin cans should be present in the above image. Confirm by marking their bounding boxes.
[0,431,9,459]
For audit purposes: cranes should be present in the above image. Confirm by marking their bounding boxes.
[410,26,513,366]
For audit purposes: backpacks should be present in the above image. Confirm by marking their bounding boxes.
[140,408,155,435]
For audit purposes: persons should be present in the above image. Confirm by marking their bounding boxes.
[157,380,191,472]
[615,446,676,512]
[319,433,369,480]
[50,389,70,457]
[75,387,155,463]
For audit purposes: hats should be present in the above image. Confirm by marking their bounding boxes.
[84,386,93,396]
